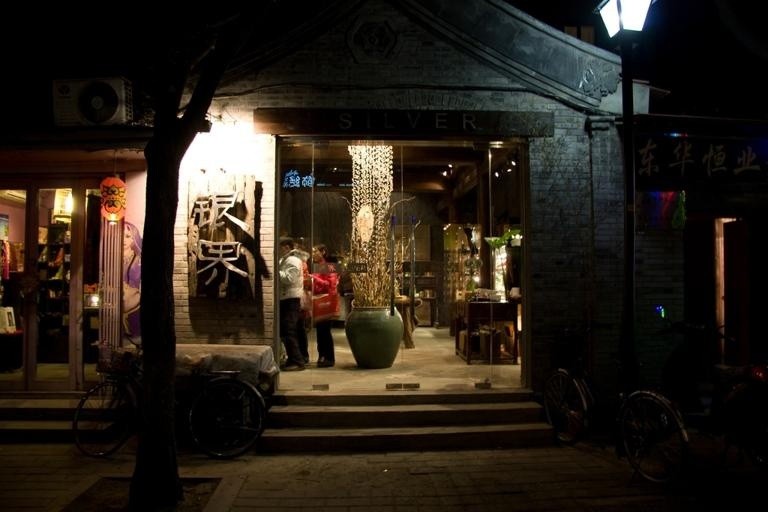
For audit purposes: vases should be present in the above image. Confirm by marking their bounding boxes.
[346,307,405,369]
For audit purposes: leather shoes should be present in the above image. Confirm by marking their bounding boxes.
[317,356,335,367]
[280,361,305,372]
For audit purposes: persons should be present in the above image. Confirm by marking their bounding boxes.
[38,224,65,298]
[278,236,337,371]
[123,221,142,349]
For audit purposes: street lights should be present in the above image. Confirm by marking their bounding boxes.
[593,0,656,392]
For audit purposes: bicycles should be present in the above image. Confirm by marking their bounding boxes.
[72,350,267,459]
[543,362,689,488]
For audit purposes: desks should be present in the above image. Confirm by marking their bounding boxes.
[455,301,522,364]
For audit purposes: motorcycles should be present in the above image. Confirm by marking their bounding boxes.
[654,304,768,455]
